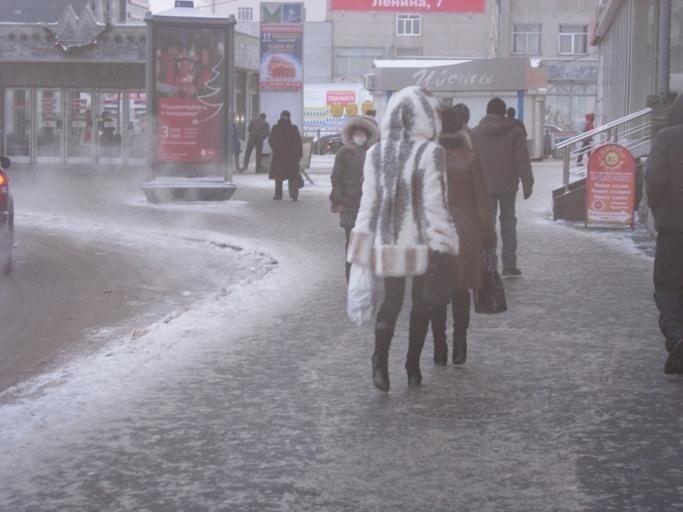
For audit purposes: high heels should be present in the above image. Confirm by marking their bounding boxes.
[405,359,422,386]
[372,353,390,392]
[453,328,466,363]
[434,335,447,366]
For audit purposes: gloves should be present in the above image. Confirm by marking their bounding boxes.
[524,186,532,200]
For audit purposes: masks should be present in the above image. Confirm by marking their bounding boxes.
[352,136,367,145]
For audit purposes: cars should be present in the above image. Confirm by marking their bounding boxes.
[0,154,14,276]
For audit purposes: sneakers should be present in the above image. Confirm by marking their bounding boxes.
[273,196,282,200]
[664,339,682,373]
[293,197,297,200]
[503,265,520,276]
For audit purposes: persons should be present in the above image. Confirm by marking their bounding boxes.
[467,97,534,276]
[99,111,116,147]
[431,106,499,367]
[240,113,270,173]
[80,111,92,145]
[505,107,527,141]
[644,91,683,375]
[575,113,596,168]
[267,110,303,202]
[231,113,244,173]
[329,114,381,285]
[450,102,473,140]
[347,87,461,394]
[165,44,211,83]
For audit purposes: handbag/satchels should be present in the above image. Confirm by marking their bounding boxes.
[347,262,377,326]
[474,267,507,313]
